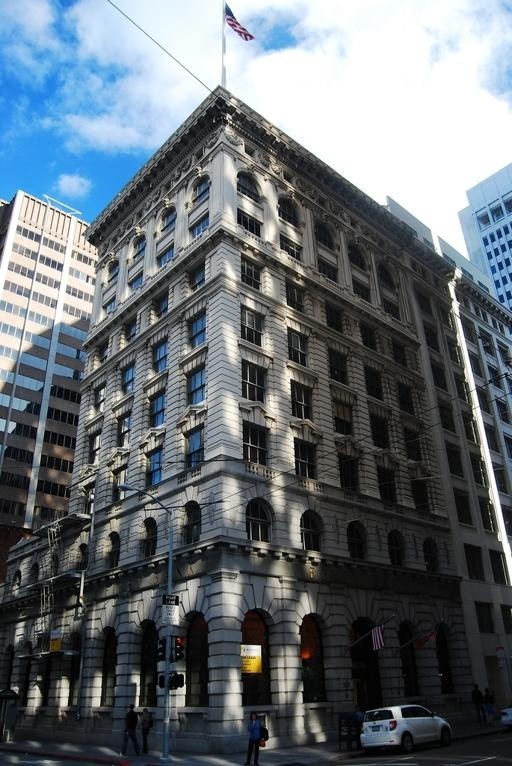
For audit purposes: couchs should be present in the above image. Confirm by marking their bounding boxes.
[174,636,185,662]
[157,639,168,661]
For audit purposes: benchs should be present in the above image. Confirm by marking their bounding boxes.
[224,1,256,42]
[415,623,441,649]
[370,624,386,654]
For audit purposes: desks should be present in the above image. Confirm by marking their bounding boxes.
[116,483,176,762]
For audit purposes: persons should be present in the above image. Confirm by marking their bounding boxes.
[119,703,141,756]
[136,707,153,755]
[351,703,365,748]
[471,684,487,725]
[244,712,262,765]
[483,688,497,725]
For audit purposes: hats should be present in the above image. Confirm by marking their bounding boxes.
[359,705,453,751]
[499,703,512,728]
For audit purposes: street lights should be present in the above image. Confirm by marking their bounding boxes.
[259,739,265,746]
